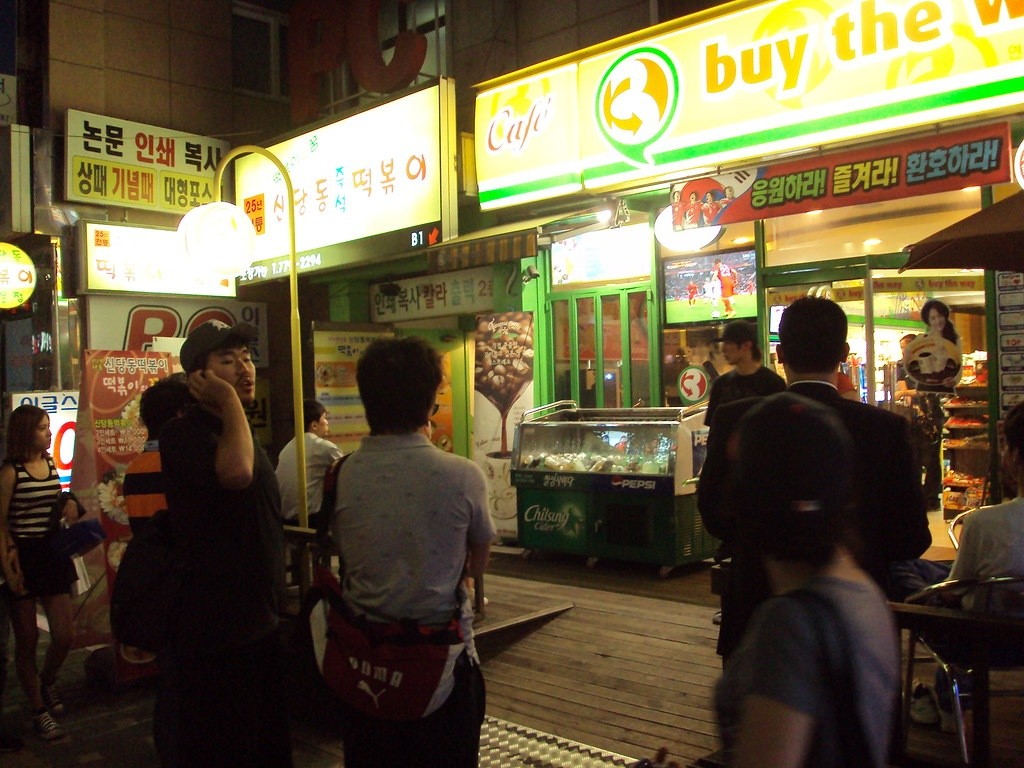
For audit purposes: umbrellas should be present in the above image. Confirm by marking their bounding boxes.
[898,188,1024,275]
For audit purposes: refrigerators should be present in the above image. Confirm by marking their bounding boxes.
[510,399,722,577]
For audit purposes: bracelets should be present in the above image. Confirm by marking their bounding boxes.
[7,545,15,552]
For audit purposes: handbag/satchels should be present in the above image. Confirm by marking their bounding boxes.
[50,514,108,561]
[323,597,464,719]
[112,506,193,652]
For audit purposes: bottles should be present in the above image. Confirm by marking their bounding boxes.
[523,451,669,474]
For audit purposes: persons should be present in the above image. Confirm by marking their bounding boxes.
[124,377,195,768]
[664,348,693,407]
[0,405,78,751]
[704,320,787,427]
[322,335,497,768]
[698,297,933,668]
[653,394,898,768]
[672,186,735,232]
[896,400,1024,732]
[159,320,294,768]
[687,258,755,319]
[275,399,344,573]
[897,333,946,512]
[916,299,963,392]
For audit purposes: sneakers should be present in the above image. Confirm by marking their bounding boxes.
[933,695,967,733]
[32,708,64,738]
[39,680,63,714]
[902,676,939,725]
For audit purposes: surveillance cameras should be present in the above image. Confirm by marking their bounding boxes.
[528,266,539,279]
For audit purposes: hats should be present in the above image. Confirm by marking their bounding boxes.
[180,319,259,374]
[713,319,757,344]
[729,390,850,509]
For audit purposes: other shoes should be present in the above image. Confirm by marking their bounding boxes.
[0,724,23,751]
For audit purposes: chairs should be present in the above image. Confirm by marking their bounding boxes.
[900,574,1023,768]
[949,505,993,548]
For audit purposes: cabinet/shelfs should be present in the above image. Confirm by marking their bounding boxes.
[942,380,994,518]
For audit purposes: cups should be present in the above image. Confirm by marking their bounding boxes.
[917,350,936,374]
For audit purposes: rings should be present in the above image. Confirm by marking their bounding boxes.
[194,385,199,391]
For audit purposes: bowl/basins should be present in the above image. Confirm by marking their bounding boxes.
[903,336,963,388]
